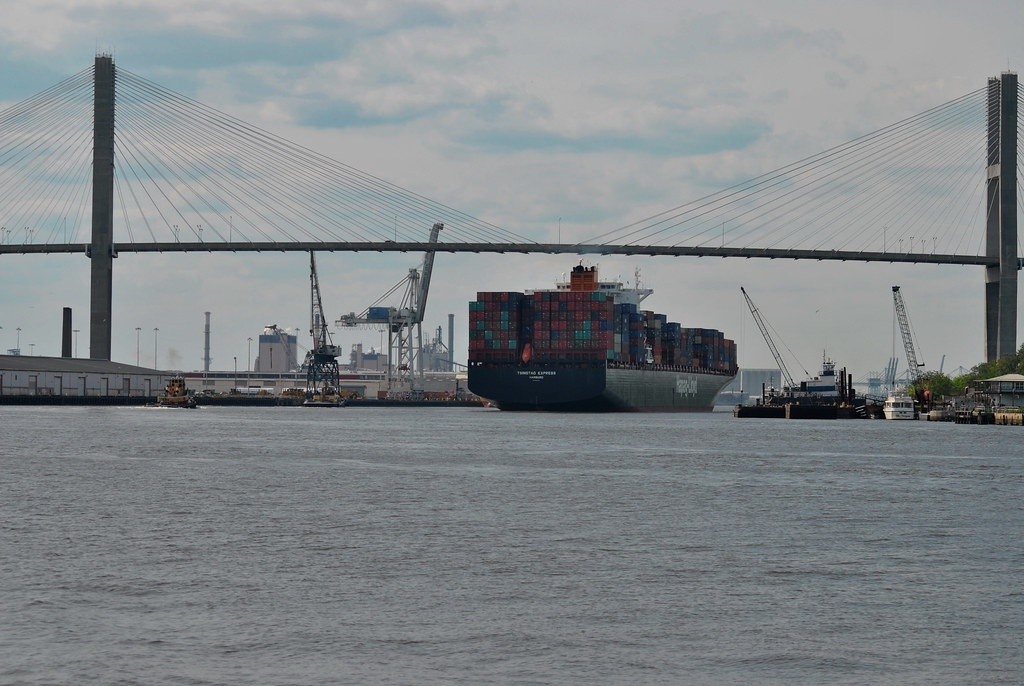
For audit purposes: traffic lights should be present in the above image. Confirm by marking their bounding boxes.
[965,387,969,394]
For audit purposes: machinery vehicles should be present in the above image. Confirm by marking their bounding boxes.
[197,387,359,401]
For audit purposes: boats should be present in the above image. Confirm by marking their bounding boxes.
[926,405,947,421]
[301,399,344,406]
[144,371,196,410]
[734,349,868,420]
[882,393,915,420]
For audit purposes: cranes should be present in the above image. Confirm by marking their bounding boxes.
[740,287,802,390]
[302,251,343,396]
[891,287,927,384]
[938,353,945,374]
[267,323,301,372]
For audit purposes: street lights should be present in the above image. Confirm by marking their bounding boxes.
[247,336,254,393]
[153,327,159,371]
[134,327,142,367]
[378,329,386,356]
[73,328,80,359]
[16,327,21,349]
[234,356,237,390]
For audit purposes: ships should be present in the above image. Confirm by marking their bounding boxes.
[466,259,739,412]
